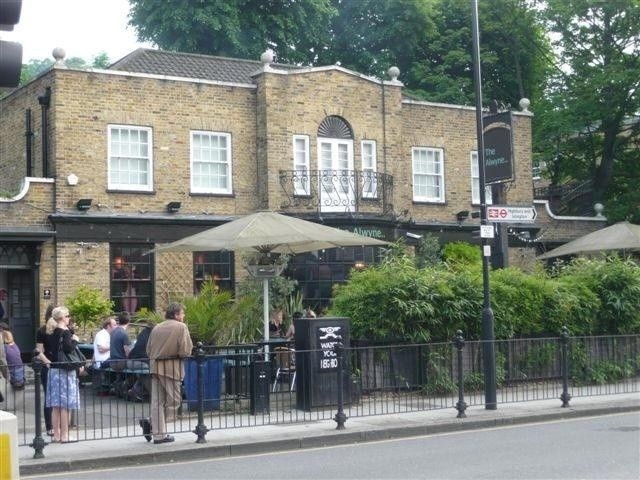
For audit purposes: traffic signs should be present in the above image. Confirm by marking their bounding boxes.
[485,204,538,225]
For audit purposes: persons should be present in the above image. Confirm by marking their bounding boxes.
[269,305,324,383]
[0,289,194,445]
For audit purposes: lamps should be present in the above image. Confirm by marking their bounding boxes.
[456,210,470,219]
[77,198,93,209]
[167,202,181,212]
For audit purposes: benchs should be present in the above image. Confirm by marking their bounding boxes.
[86,364,149,375]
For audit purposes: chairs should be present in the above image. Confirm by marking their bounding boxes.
[272,347,296,394]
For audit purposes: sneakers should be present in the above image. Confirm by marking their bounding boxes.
[109,384,144,402]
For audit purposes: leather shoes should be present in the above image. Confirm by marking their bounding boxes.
[139,419,152,441]
[154,435,174,444]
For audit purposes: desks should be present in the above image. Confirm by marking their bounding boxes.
[78,343,94,349]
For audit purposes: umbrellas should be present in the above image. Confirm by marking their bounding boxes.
[538,222,640,260]
[141,209,392,360]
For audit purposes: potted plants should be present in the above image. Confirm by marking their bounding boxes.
[132,277,260,411]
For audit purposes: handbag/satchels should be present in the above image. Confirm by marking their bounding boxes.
[58,337,86,370]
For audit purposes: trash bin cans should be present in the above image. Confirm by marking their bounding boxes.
[183,354,222,412]
[225,365,251,395]
